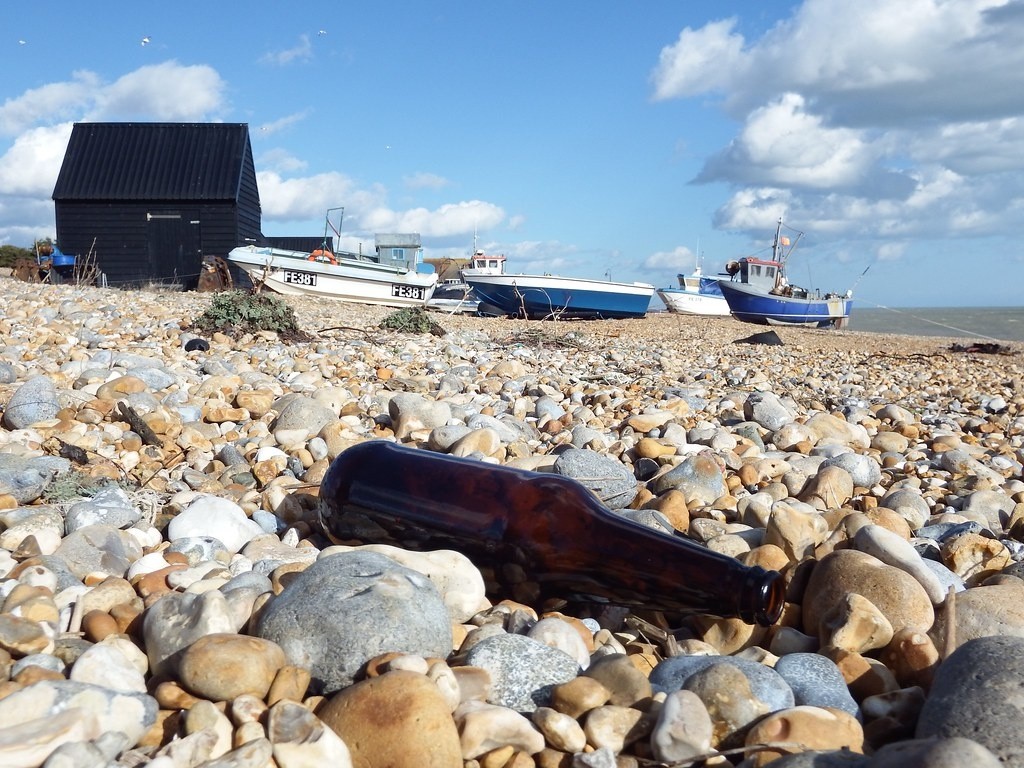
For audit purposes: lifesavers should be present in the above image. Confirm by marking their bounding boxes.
[308,250,339,266]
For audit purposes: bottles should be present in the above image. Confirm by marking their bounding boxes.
[316,438,787,628]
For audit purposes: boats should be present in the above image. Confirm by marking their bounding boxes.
[718,217,854,327]
[462,270,654,321]
[227,244,439,308]
[656,267,741,317]
[429,255,507,312]
